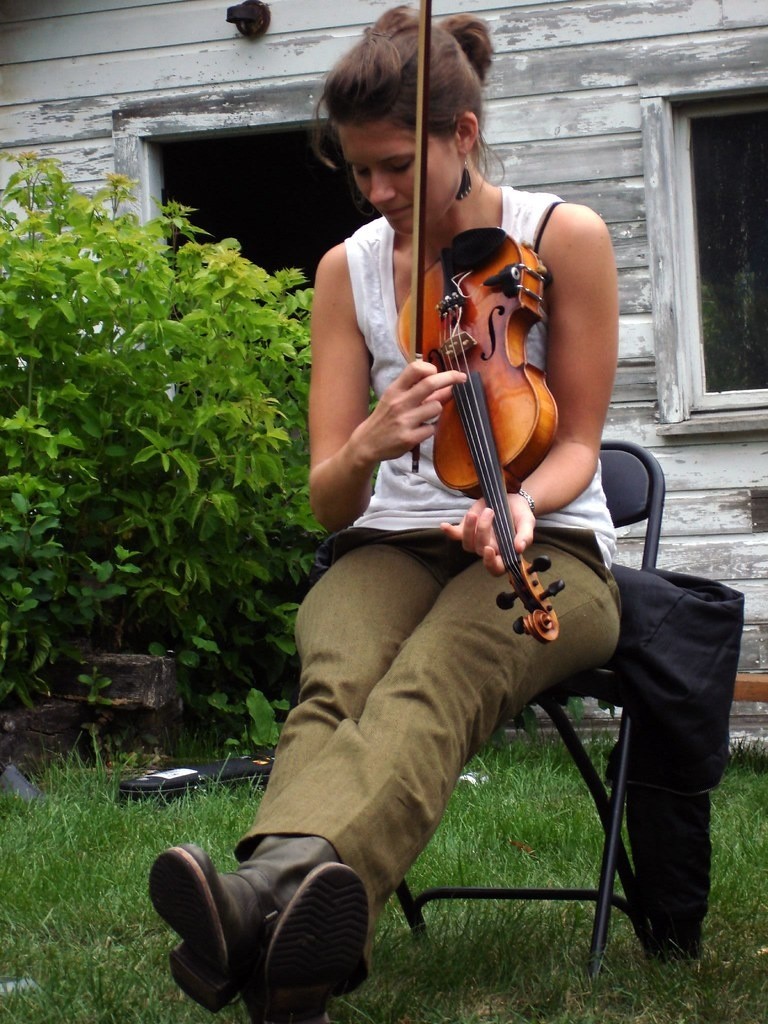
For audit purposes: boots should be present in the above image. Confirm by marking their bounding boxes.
[238,863,369,1024]
[147,833,343,1014]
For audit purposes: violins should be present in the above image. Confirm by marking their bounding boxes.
[395,225,569,645]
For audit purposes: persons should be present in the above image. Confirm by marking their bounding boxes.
[146,6,620,1023]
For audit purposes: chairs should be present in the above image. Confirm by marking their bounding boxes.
[391,436,667,986]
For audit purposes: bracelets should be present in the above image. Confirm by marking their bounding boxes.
[519,488,537,512]
